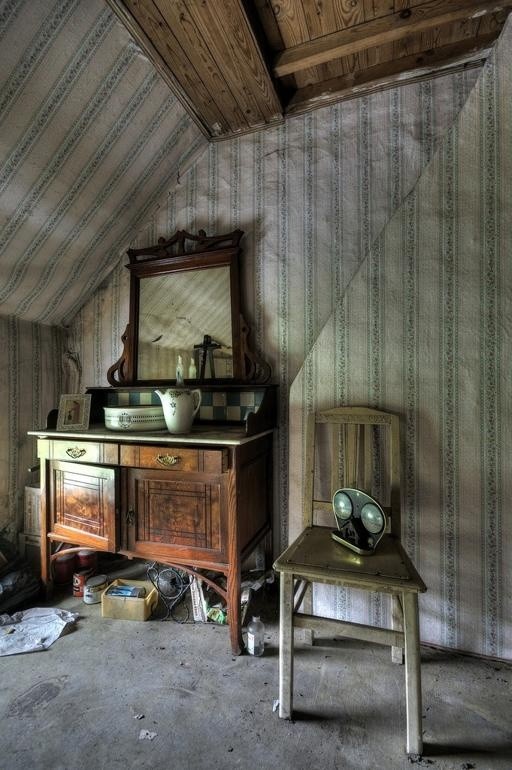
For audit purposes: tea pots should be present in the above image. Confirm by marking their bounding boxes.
[154,388,202,434]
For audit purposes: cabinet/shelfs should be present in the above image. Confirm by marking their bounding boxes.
[27,424,274,655]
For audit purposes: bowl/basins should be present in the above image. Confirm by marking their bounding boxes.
[102,405,167,432]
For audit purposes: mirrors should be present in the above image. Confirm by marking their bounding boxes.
[107,229,271,387]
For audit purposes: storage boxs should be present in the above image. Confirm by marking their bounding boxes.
[101,579,158,621]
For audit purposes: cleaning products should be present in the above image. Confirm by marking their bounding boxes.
[175,354,184,386]
[188,359,196,378]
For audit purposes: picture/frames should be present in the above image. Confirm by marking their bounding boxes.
[56,394,91,431]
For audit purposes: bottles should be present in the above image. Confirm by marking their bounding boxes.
[246,615,265,657]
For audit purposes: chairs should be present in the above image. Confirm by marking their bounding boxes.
[273,406,427,755]
